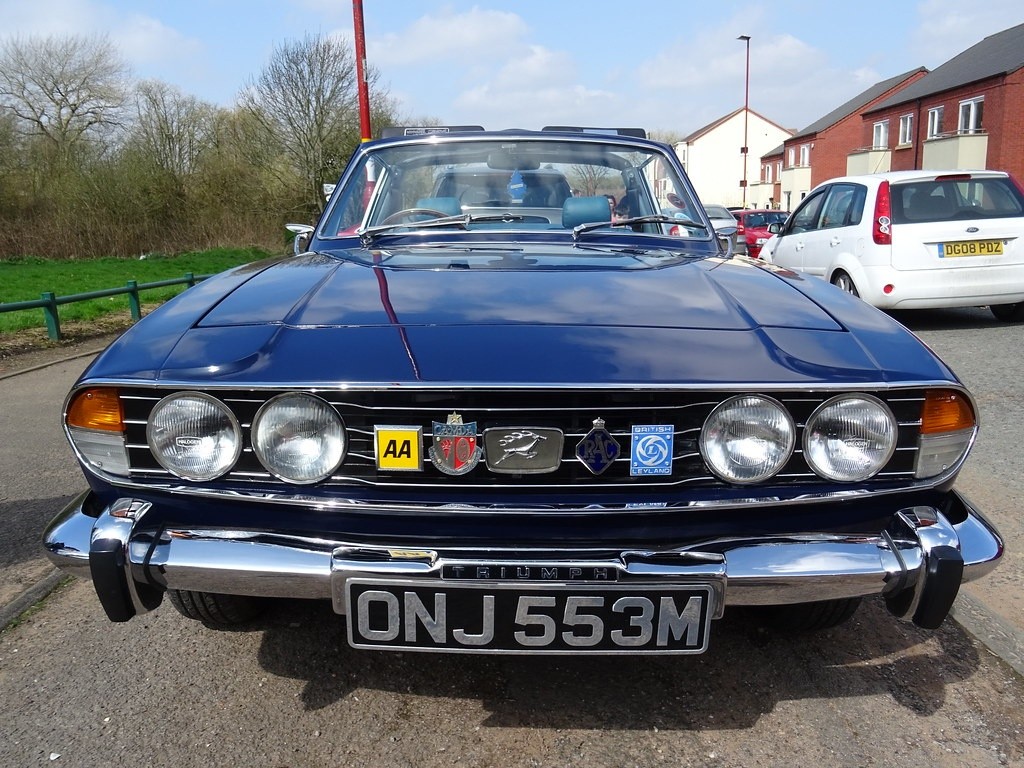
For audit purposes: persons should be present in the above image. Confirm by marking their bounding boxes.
[603,194,633,231]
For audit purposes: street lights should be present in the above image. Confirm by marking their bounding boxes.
[737,35,750,209]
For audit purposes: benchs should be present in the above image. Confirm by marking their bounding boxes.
[918,195,950,219]
[391,223,632,233]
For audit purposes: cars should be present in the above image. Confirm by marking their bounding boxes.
[662,204,746,255]
[43,125,1005,655]
[737,209,791,259]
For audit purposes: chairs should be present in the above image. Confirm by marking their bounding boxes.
[909,193,929,211]
[828,193,865,225]
[415,196,461,220]
[562,196,611,229]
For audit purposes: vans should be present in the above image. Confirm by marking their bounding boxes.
[758,170,1024,320]
